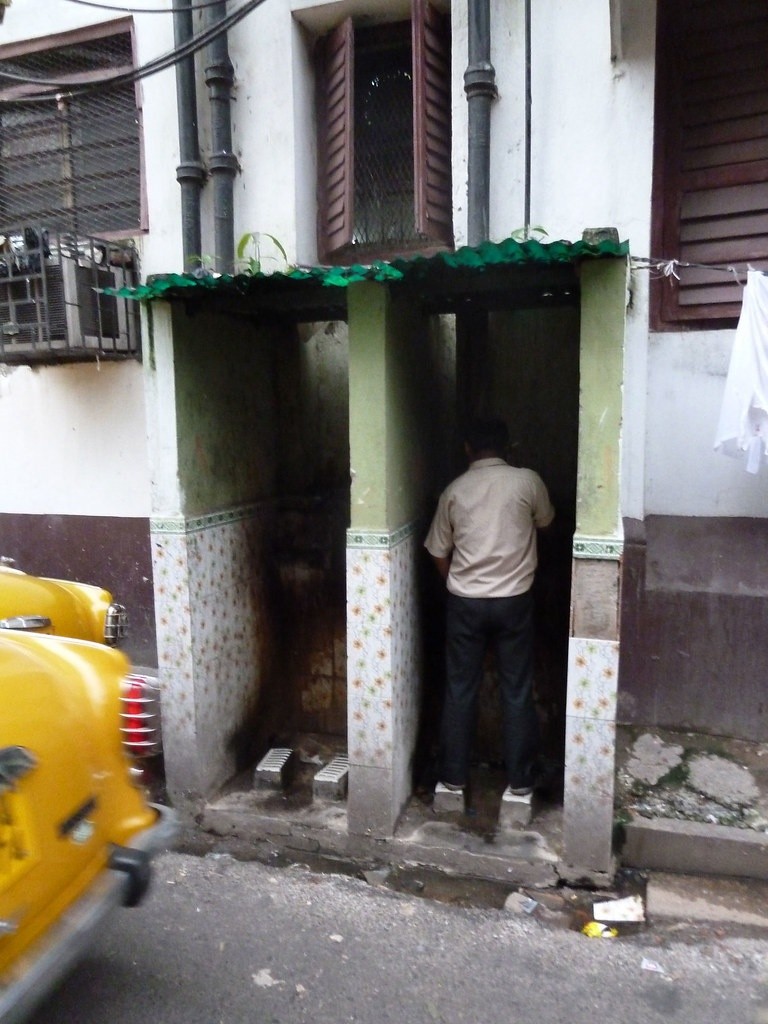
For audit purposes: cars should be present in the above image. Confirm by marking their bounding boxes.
[0,555,176,1024]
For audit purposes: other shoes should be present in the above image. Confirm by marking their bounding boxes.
[437,766,471,790]
[511,761,541,796]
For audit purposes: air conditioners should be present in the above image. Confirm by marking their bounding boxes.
[0,255,136,352]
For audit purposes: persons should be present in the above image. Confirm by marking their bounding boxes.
[424,419,556,795]
